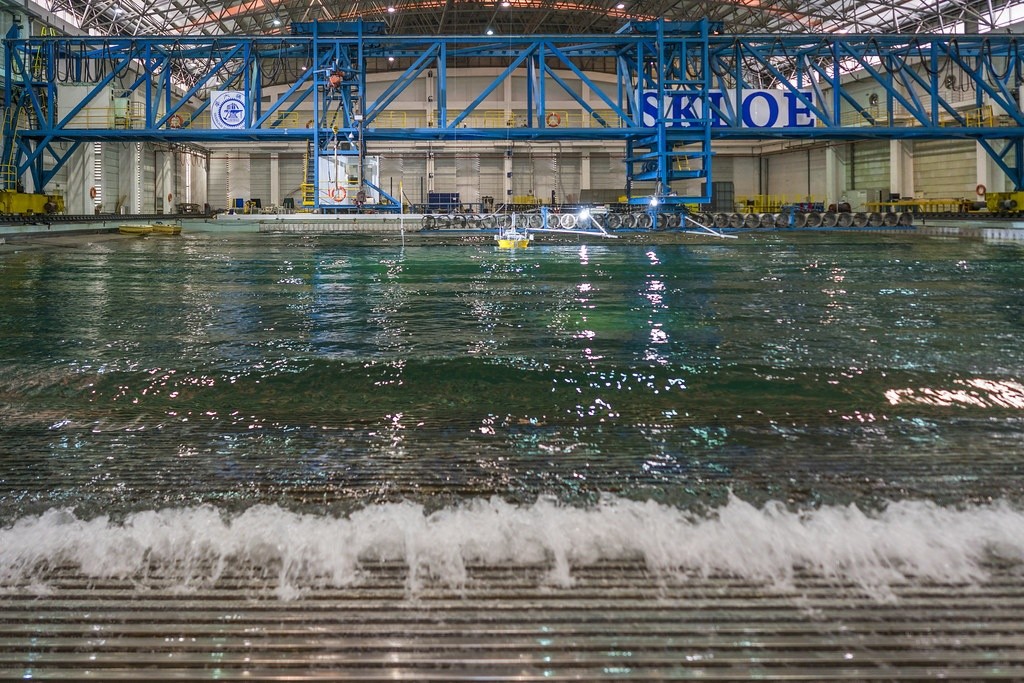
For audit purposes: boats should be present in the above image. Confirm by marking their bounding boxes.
[119,224,153,236]
[497,238,531,250]
[151,224,183,235]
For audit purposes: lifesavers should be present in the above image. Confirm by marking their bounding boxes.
[975,184,986,195]
[167,193,173,202]
[545,112,562,127]
[168,115,184,128]
[90,188,97,198]
[306,119,315,128]
[331,186,347,201]
